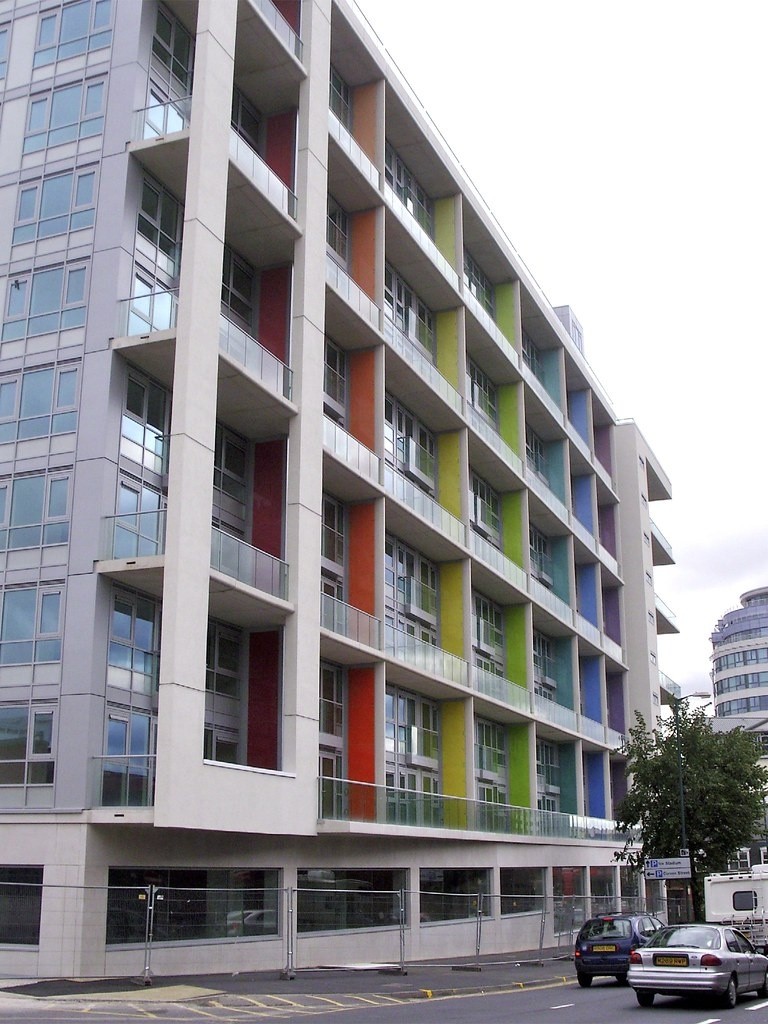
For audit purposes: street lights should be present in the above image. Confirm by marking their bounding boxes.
[675,692,711,923]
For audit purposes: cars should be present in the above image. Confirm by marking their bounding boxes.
[626,924,768,1009]
[168,918,218,941]
[325,914,374,931]
[106,908,170,944]
[221,909,277,936]
[377,910,430,926]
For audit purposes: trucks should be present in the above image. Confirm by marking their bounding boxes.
[704,864,768,957]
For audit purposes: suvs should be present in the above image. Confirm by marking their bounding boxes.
[575,911,666,988]
